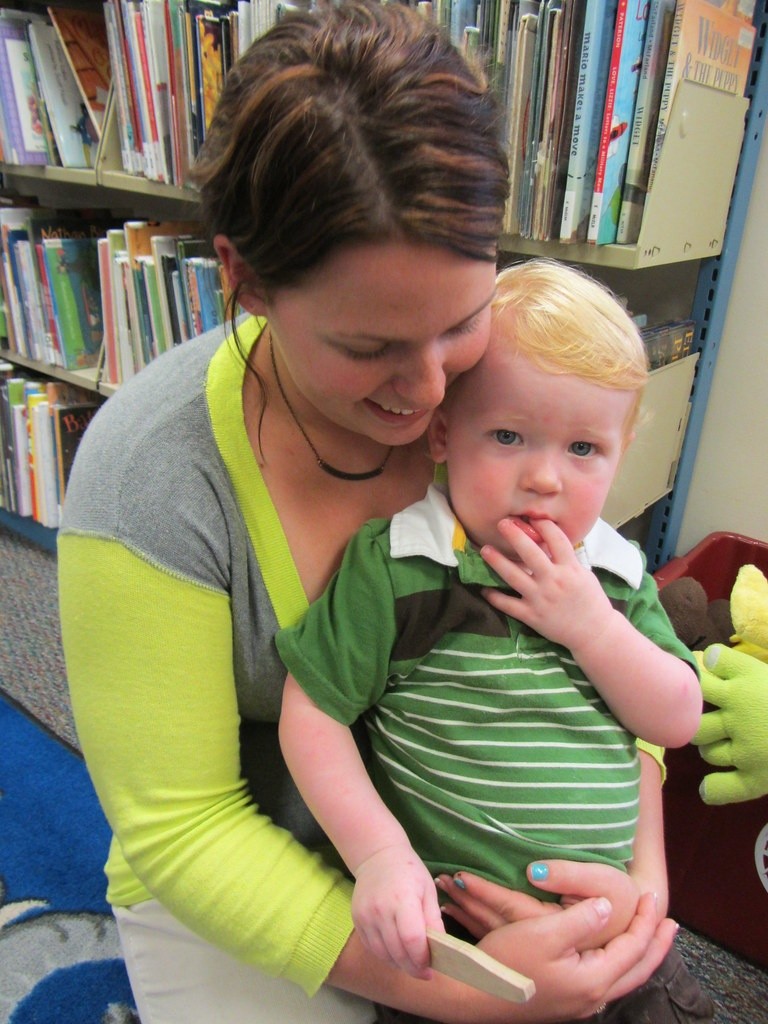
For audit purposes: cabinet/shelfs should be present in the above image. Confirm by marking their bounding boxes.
[0,0,768,574]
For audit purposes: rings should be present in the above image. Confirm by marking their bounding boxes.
[596,1004,607,1015]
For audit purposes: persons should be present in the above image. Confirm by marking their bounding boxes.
[56,3,678,1024]
[272,260,715,1024]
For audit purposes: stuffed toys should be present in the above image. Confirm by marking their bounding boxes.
[656,563,768,805]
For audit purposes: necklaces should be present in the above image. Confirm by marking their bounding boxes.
[267,328,394,481]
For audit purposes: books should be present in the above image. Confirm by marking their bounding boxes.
[1,1,758,529]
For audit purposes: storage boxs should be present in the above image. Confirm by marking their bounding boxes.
[655,532,768,971]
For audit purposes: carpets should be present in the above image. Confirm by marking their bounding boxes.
[0,689,141,1024]
